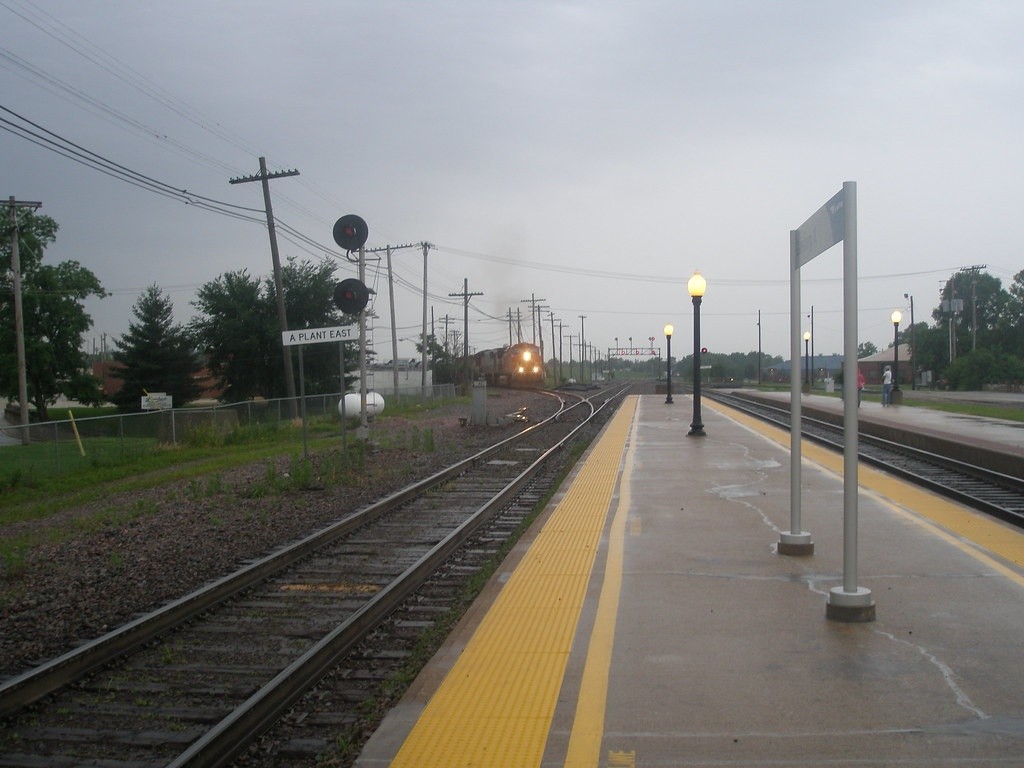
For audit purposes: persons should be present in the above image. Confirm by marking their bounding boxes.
[882,365,891,407]
[858,370,865,408]
[840,370,844,399]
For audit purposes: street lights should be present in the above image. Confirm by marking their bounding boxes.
[891,311,901,390]
[662,325,675,404]
[687,269,709,436]
[803,331,810,393]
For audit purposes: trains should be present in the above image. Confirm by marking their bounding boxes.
[366,343,542,390]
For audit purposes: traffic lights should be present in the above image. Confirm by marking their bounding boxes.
[652,351,655,355]
[702,348,707,353]
[334,278,368,315]
[332,214,368,250]
[621,351,625,355]
[635,351,639,355]
